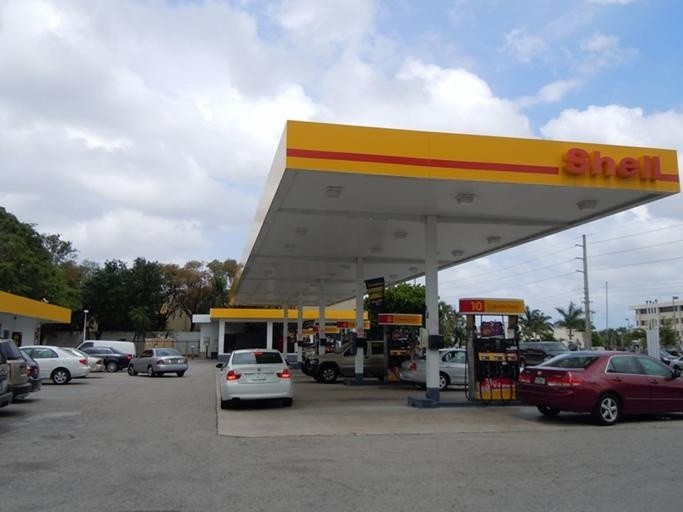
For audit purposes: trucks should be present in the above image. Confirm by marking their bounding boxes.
[75,339,137,356]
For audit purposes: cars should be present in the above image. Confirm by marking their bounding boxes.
[79,346,135,375]
[125,347,190,378]
[397,345,468,392]
[515,350,682,427]
[612,345,682,371]
[18,346,42,393]
[215,348,295,409]
[16,344,93,385]
[59,346,106,373]
[0,343,13,409]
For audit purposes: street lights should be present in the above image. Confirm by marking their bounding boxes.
[82,309,89,342]
[671,296,679,347]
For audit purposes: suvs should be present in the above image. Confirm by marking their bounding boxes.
[300,339,383,383]
[0,337,33,397]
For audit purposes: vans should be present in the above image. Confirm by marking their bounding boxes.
[514,339,570,368]
